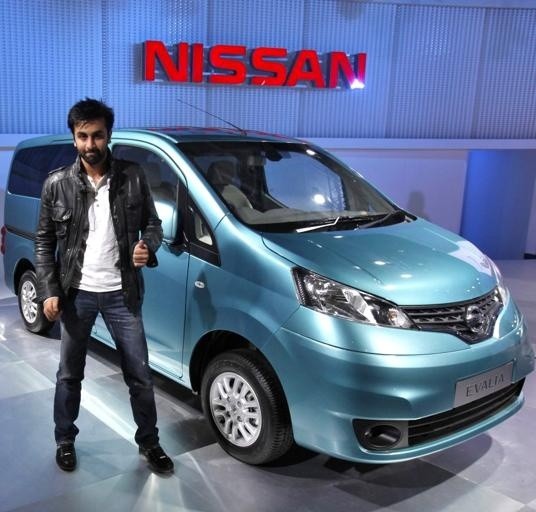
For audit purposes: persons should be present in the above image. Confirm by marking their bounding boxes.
[33,94,175,475]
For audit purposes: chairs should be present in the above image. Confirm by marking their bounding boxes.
[207,161,253,210]
[143,160,176,240]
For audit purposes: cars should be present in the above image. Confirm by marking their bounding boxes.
[0,98,536,468]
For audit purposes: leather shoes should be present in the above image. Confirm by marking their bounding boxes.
[138,445,174,472]
[55,444,77,470]
